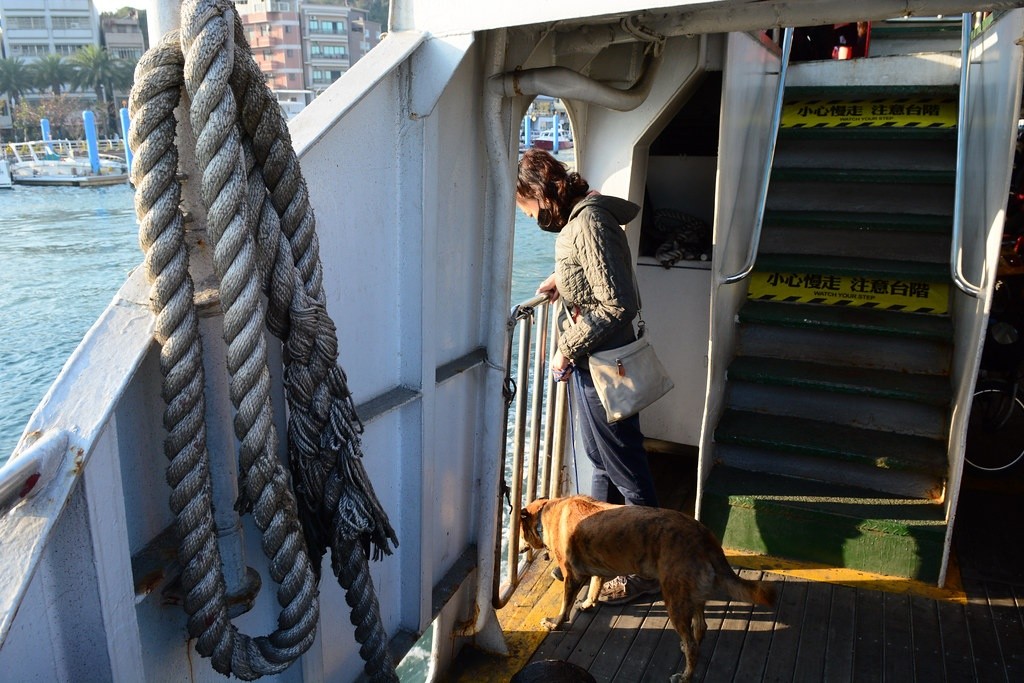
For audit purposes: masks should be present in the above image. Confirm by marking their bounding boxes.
[537,199,561,232]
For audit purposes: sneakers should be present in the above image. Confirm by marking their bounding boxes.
[596,575,662,604]
[551,566,565,582]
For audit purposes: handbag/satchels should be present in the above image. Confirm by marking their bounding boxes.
[588,337,674,424]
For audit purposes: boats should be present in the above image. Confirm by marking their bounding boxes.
[532,127,573,151]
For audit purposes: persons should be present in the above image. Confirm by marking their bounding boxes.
[516,148,664,608]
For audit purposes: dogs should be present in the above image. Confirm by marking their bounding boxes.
[520,494,776,683]
